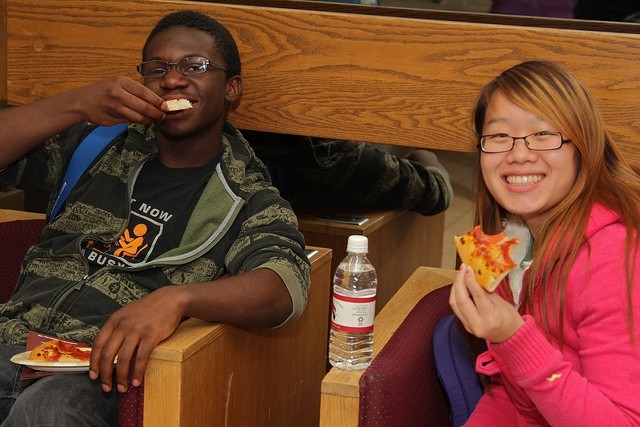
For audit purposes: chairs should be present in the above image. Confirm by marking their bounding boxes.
[0,208,334,426]
[294,202,445,317]
[320,262,467,426]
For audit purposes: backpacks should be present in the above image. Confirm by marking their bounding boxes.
[432,313,492,426]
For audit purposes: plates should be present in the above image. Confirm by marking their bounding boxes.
[10,347,118,372]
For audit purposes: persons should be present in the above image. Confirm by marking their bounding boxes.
[238,131,454,220]
[1,11,310,426]
[448,59,640,426]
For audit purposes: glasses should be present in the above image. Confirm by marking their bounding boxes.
[137,55,228,79]
[476,130,572,153]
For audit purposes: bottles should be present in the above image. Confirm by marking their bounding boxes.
[328,235,377,371]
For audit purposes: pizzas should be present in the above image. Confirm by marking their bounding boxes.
[28,337,92,363]
[452,224,521,291]
[164,98,195,112]
